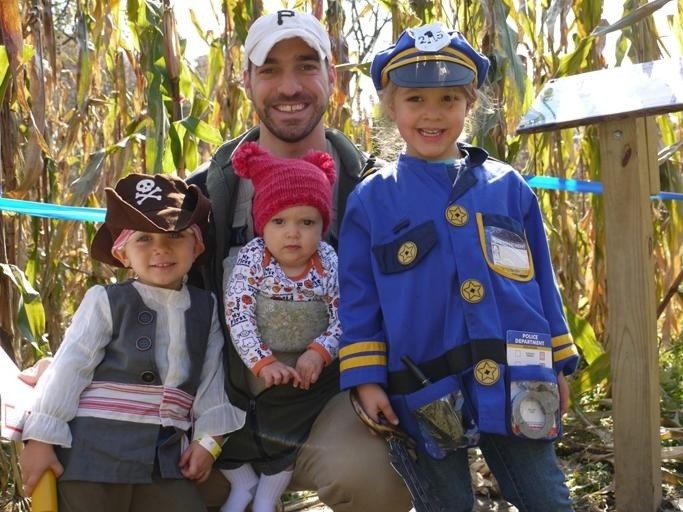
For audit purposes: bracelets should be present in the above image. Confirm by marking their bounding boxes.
[195,435,222,461]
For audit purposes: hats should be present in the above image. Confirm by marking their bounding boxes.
[370,22,491,95]
[91,172,214,270]
[229,137,338,235]
[241,8,333,72]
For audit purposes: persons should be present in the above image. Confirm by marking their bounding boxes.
[17,170,250,512]
[338,20,590,511]
[14,6,411,512]
[210,141,341,512]
